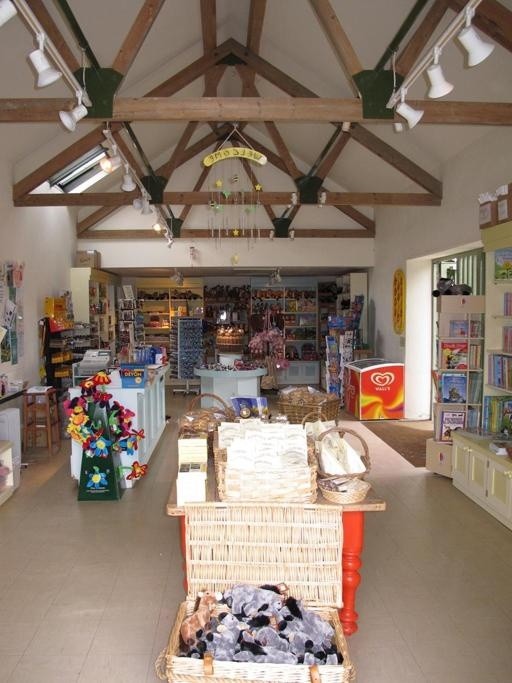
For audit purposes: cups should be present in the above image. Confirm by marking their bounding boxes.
[354,328,363,350]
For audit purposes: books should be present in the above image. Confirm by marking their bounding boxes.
[431,293,512,443]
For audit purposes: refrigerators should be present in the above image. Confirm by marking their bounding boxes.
[343,358,405,422]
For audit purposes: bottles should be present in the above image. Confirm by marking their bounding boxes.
[17,375,24,391]
[50,335,93,378]
[132,345,155,365]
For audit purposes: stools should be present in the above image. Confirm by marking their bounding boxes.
[21,384,63,462]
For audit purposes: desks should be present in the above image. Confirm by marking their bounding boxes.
[164,450,385,638]
[1,384,28,508]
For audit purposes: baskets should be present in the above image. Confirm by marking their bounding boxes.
[303,408,373,504]
[154,587,357,683]
[177,392,239,441]
[277,383,344,424]
[210,425,320,505]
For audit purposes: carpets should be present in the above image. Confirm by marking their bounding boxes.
[362,416,433,468]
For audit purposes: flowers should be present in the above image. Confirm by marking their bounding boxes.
[61,366,149,491]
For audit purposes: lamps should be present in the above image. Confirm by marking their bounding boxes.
[387,0,498,128]
[96,128,177,252]
[1,0,91,137]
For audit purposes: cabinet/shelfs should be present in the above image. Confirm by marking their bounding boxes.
[477,222,512,450]
[424,291,485,482]
[450,426,511,530]
[41,271,366,402]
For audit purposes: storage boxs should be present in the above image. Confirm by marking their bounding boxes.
[76,250,103,269]
[493,183,512,223]
[479,192,498,230]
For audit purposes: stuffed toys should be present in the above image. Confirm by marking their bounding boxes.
[178,581,340,667]
